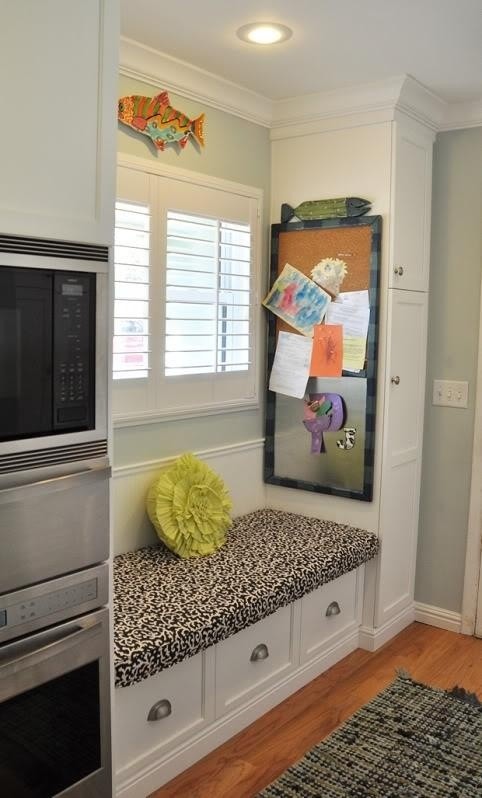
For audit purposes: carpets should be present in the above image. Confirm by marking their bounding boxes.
[251,661,481,798]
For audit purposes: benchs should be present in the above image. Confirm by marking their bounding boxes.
[110,509,377,798]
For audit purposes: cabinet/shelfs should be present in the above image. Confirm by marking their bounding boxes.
[112,561,367,793]
[269,104,440,651]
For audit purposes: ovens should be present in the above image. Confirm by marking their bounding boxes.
[1,456,113,596]
[1,252,110,457]
[1,606,113,796]
